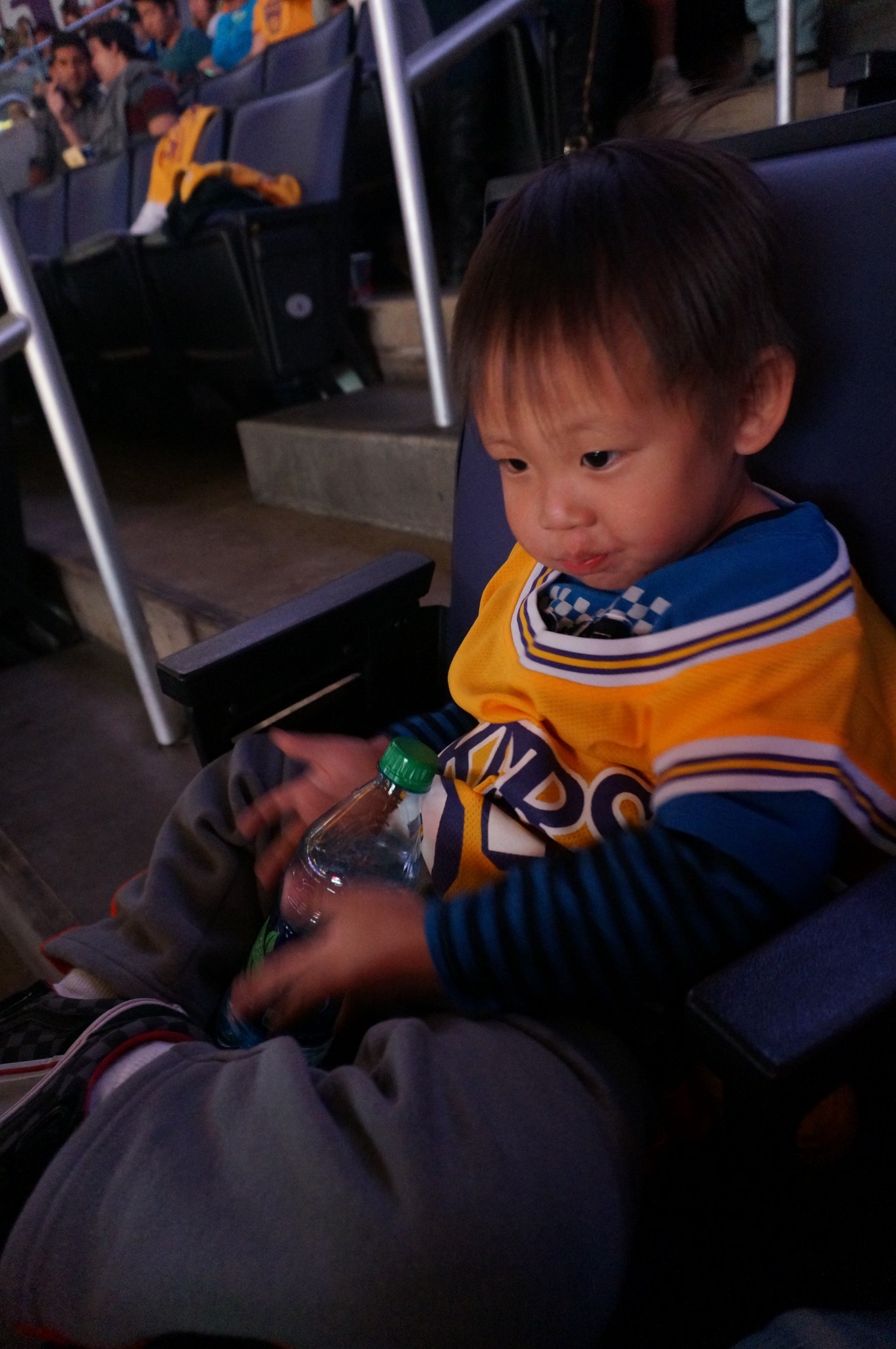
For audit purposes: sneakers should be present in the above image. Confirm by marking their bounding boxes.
[0,998,205,1263]
[0,978,132,1119]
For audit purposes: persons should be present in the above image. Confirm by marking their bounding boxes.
[0,0,772,179]
[0,129,896,1349]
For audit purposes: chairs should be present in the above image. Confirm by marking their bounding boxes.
[2,0,457,482]
[152,98,896,1349]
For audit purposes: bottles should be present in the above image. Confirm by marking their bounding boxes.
[217,737,439,1068]
